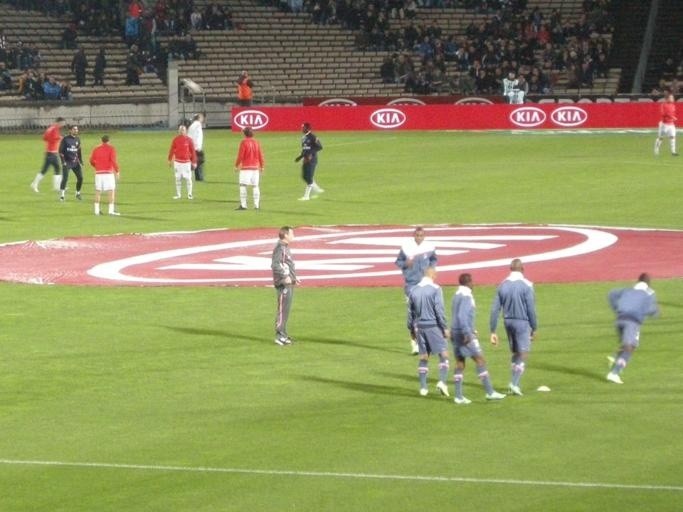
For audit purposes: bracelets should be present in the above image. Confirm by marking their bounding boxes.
[61,156,66,163]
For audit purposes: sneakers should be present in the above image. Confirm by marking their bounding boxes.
[485,391,507,400]
[53,186,68,192]
[187,194,193,200]
[30,182,39,193]
[410,340,419,353]
[275,336,291,346]
[59,195,64,203]
[419,387,428,397]
[95,211,100,215]
[606,370,624,383]
[508,383,514,395]
[436,381,449,397]
[74,193,82,201]
[172,195,182,199]
[235,206,247,211]
[607,356,615,367]
[108,212,120,216]
[511,384,522,395]
[453,396,472,404]
[298,195,309,202]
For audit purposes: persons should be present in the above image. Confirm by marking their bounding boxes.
[89,136,120,216]
[654,93,680,156]
[187,113,205,181]
[236,70,254,106]
[407,267,450,397]
[30,117,70,193]
[490,259,538,396]
[295,122,324,201]
[450,274,506,404]
[286,2,682,105]
[1,1,233,100]
[234,126,265,210]
[272,226,297,345]
[167,125,197,200]
[395,227,438,355]
[607,273,658,384]
[59,125,84,201]
